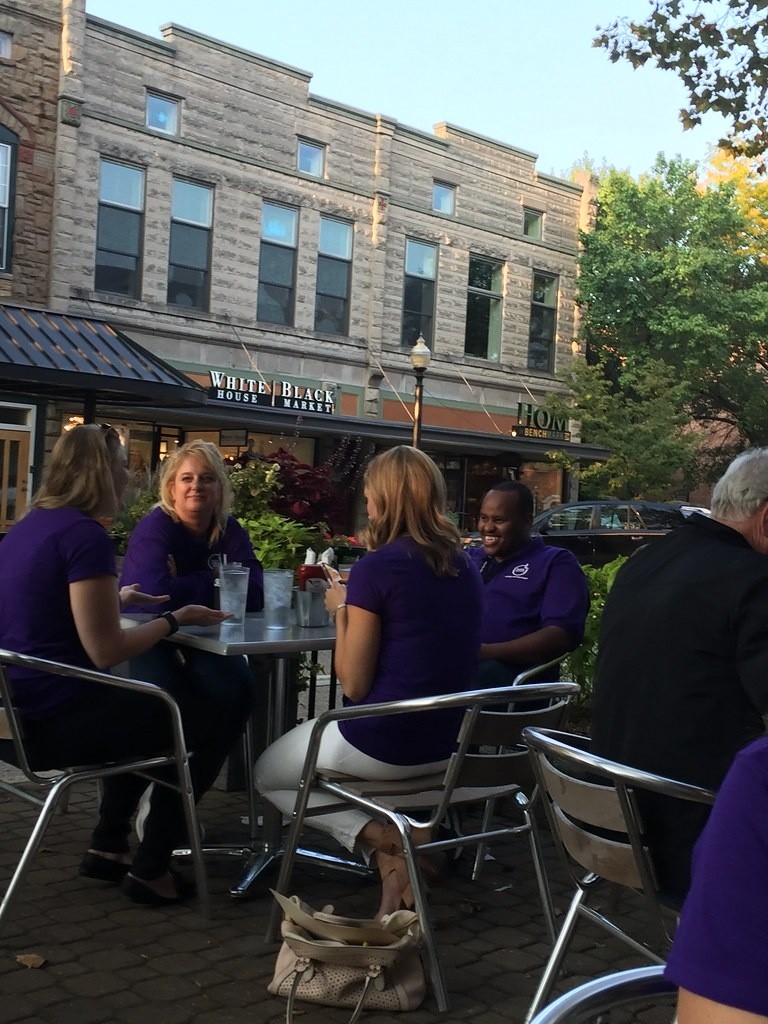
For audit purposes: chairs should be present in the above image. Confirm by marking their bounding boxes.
[517,727,720,1024]
[467,645,571,885]
[263,682,584,1015]
[0,648,213,926]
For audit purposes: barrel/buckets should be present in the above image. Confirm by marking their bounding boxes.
[291,585,331,627]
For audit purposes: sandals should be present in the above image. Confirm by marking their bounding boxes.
[373,825,449,922]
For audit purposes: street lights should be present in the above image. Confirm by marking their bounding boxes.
[410,332,431,452]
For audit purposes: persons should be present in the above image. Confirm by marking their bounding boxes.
[116,440,264,618]
[457,481,591,726]
[250,444,487,921]
[659,739,768,1022]
[588,448,768,903]
[0,424,251,907]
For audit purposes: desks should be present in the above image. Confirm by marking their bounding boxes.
[118,607,387,904]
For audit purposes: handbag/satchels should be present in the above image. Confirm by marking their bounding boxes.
[266,885,426,1024]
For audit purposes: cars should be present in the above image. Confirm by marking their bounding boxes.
[459,500,705,570]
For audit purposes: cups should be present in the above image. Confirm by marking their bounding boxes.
[220,565,250,626]
[263,568,295,629]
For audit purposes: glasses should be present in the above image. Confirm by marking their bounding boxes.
[97,421,120,444]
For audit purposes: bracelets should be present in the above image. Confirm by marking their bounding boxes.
[156,609,180,637]
[330,603,347,623]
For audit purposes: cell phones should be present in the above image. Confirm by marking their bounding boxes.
[321,562,345,589]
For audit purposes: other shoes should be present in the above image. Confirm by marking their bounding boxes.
[121,870,197,907]
[79,851,135,887]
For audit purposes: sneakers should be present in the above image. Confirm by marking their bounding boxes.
[429,805,463,862]
